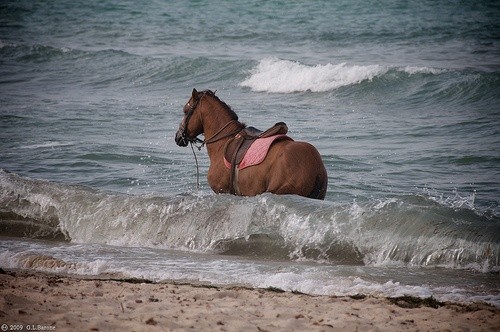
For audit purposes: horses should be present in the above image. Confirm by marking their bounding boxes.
[174,88,329,200]
